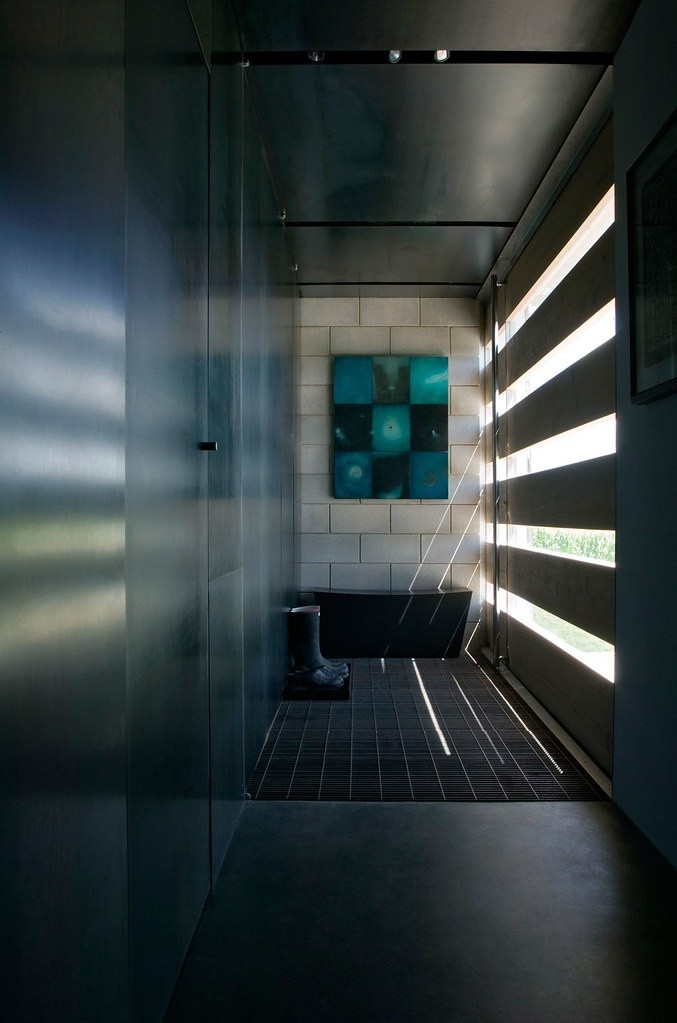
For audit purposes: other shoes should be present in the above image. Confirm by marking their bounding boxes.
[287,669,343,692]
[317,665,350,681]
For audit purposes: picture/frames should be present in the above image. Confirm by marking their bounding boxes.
[625,107,677,406]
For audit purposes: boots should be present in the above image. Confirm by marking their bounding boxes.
[292,604,348,673]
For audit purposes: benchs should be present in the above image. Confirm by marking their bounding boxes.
[312,587,473,659]
